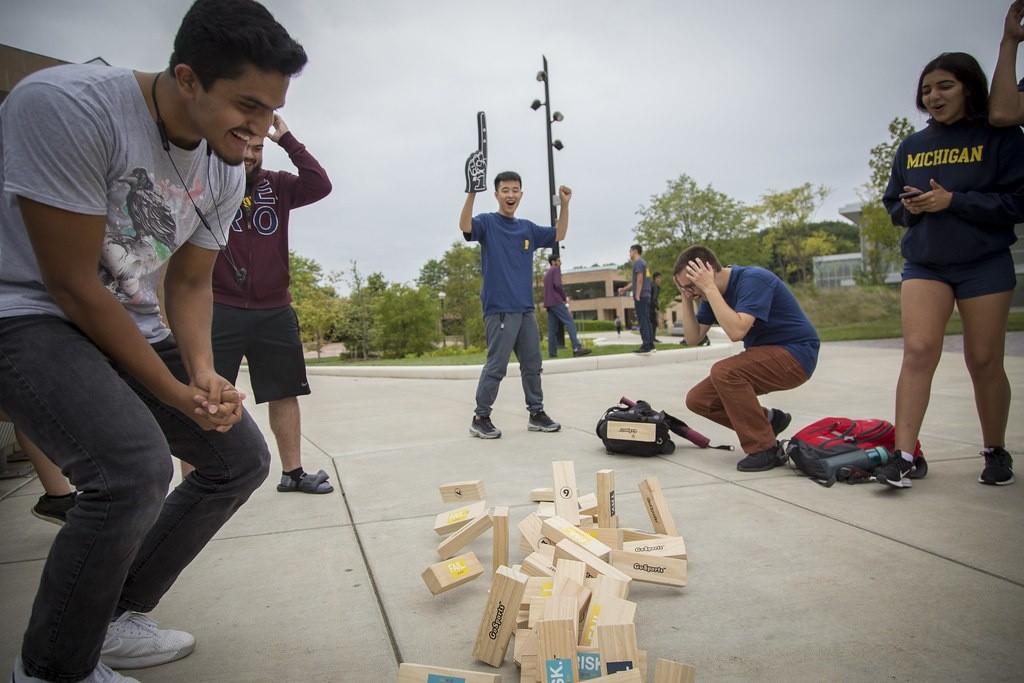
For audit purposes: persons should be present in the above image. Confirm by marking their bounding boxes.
[0,0,308,683]
[181,115,333,493]
[672,245,820,472]
[987,0,1024,127]
[615,316,621,334]
[618,244,655,353]
[460,172,571,438]
[543,254,592,357]
[651,272,662,342]
[875,51,1024,488]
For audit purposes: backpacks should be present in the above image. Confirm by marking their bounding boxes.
[776,417,928,488]
[596,400,687,457]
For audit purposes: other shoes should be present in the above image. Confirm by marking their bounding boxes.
[633,345,657,356]
[573,348,592,357]
[654,339,661,343]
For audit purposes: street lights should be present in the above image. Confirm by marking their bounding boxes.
[531,55,566,348]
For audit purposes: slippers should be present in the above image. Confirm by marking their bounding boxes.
[277,467,334,494]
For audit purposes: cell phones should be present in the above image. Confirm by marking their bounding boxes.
[899,191,922,199]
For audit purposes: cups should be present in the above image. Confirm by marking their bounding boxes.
[823,446,888,478]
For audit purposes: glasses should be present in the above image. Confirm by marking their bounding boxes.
[681,284,695,292]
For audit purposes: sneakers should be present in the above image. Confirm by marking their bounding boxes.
[978,445,1015,485]
[100,610,196,670]
[527,411,561,432]
[876,449,913,487]
[469,415,501,439]
[31,491,79,527]
[770,408,792,439]
[737,440,787,472]
[10,647,141,683]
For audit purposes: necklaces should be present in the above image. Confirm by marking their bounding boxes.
[153,72,246,286]
